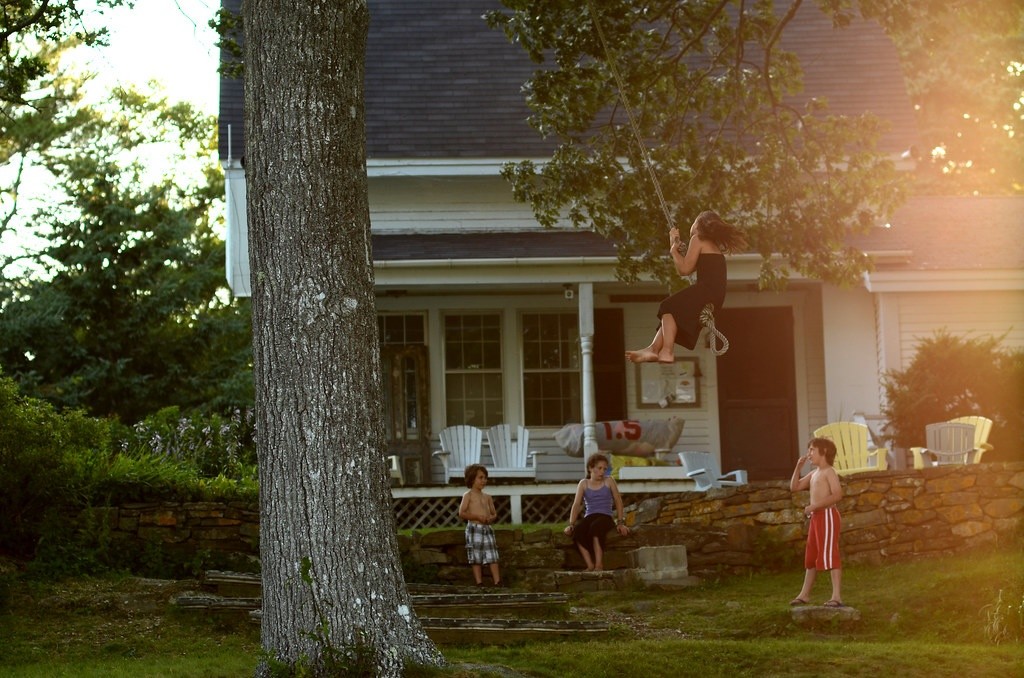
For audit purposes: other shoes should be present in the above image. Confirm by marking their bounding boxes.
[494,582,508,587]
[476,582,486,587]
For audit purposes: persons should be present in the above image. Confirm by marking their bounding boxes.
[624,210,747,364]
[459,463,510,588]
[563,455,630,572]
[789,438,843,607]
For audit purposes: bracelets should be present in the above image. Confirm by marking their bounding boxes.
[616,519,625,526]
[568,522,575,526]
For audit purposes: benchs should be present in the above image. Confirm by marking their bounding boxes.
[432,424,548,486]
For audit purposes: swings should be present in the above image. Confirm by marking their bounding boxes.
[589,0,730,356]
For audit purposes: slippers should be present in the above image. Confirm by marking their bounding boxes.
[823,600,845,607]
[789,598,808,606]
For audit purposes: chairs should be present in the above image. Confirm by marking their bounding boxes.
[813,422,888,476]
[387,455,403,486]
[678,451,748,491]
[908,416,994,469]
